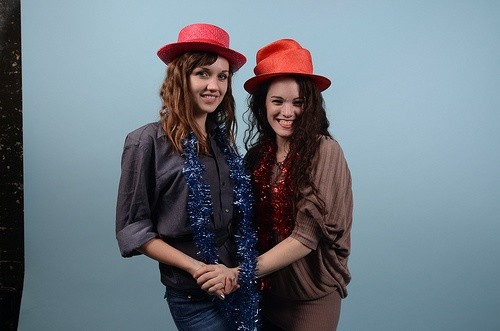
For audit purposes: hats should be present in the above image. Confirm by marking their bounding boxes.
[156,23,247,73]
[244,38,331,93]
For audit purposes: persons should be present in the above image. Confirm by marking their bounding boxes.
[192,38,354,331]
[114,24,246,331]
